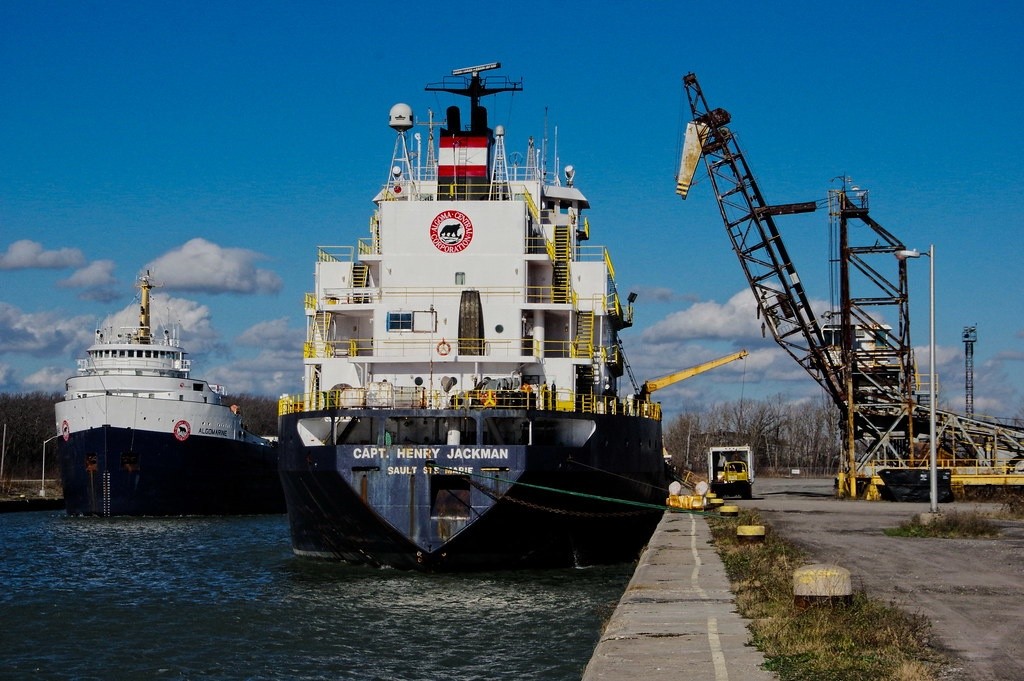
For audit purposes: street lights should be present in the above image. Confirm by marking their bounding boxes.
[894,244,938,513]
[42,436,58,490]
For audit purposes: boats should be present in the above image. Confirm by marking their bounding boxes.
[275,61,675,573]
[53,268,289,518]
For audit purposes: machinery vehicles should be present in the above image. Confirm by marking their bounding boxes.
[638,349,749,403]
